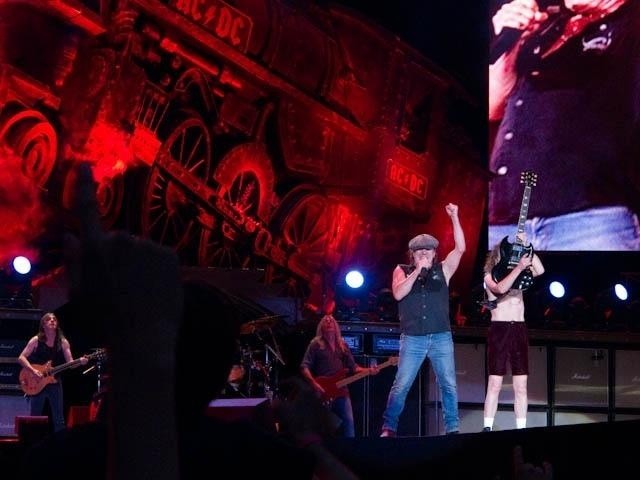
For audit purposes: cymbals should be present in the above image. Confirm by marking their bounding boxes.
[238,319,279,334]
[257,315,291,321]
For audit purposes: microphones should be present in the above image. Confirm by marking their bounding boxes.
[422,268,428,277]
[490,0,560,66]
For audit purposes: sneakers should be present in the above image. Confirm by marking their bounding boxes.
[379,429,396,438]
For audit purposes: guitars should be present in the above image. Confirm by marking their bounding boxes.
[492,170,538,291]
[311,356,399,407]
[19,348,108,396]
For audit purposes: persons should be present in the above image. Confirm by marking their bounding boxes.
[375,202,469,438]
[488,0,626,225]
[16,312,89,435]
[298,313,380,439]
[478,230,546,431]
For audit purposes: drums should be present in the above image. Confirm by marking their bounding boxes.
[226,364,246,383]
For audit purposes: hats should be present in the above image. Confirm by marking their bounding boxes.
[408,234,440,252]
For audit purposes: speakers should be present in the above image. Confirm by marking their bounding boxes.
[206,398,274,432]
